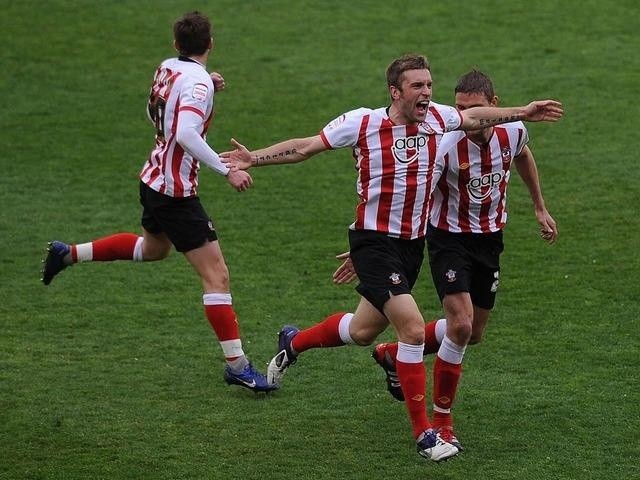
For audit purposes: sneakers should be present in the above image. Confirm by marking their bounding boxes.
[40,240,70,285]
[223,362,280,394]
[416,422,462,462]
[266,326,300,384]
[373,343,405,401]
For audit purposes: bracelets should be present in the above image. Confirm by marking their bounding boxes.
[251,150,259,168]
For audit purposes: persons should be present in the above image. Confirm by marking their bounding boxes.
[217,52,565,464]
[40,9,280,397]
[332,69,560,453]
